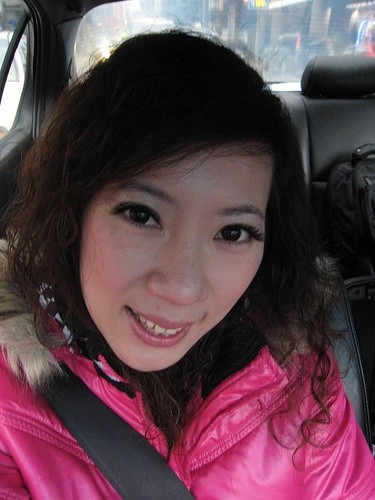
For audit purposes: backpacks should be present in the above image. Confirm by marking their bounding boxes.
[323,144,375,266]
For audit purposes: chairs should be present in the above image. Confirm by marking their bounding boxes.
[257,260,375,451]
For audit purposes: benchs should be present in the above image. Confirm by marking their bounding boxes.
[272,57,375,235]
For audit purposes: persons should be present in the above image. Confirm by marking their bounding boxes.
[1,29,375,500]
